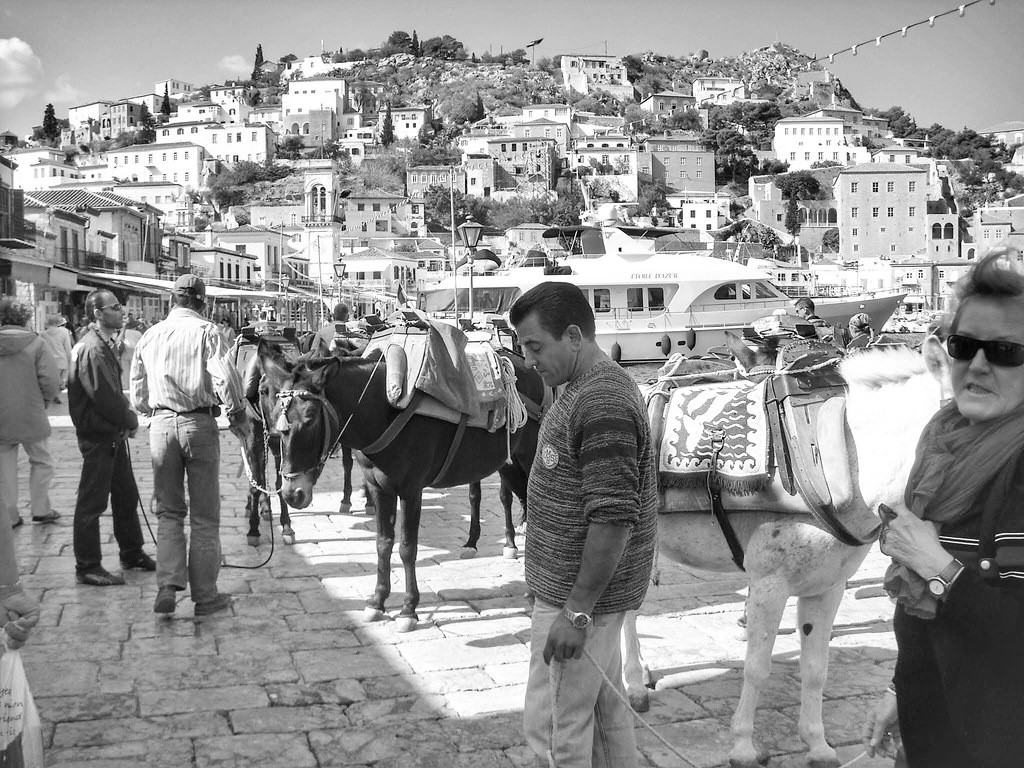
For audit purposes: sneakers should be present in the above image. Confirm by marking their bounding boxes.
[76,565,125,585]
[120,549,156,572]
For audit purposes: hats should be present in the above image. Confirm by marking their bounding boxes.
[44,314,67,327]
[171,274,206,300]
[126,321,139,329]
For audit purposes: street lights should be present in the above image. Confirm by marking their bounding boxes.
[333,257,346,303]
[458,215,484,320]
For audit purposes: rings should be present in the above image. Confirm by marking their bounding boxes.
[883,731,892,737]
[883,530,890,539]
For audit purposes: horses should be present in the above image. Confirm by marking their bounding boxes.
[632,336,954,766]
[226,324,298,546]
[269,347,561,633]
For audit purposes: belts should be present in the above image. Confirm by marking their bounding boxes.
[149,405,221,417]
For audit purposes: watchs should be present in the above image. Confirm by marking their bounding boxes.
[560,606,591,630]
[927,558,965,601]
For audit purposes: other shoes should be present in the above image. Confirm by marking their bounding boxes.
[195,593,230,615]
[155,586,176,612]
[12,517,23,529]
[32,509,62,520]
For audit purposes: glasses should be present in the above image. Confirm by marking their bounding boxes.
[100,303,122,311]
[796,305,808,314]
[947,334,1024,367]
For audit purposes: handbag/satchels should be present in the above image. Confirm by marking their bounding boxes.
[0,618,43,768]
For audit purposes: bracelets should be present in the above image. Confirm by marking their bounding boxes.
[887,684,897,696]
[0,580,22,593]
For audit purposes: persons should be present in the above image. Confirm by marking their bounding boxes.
[0,500,41,659]
[67,288,157,586]
[860,246,1023,768]
[310,303,349,358]
[43,312,236,404]
[510,282,658,767]
[846,313,873,348]
[794,298,822,321]
[130,274,247,616]
[0,296,62,530]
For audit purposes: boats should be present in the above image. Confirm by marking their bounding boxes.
[421,218,910,365]
